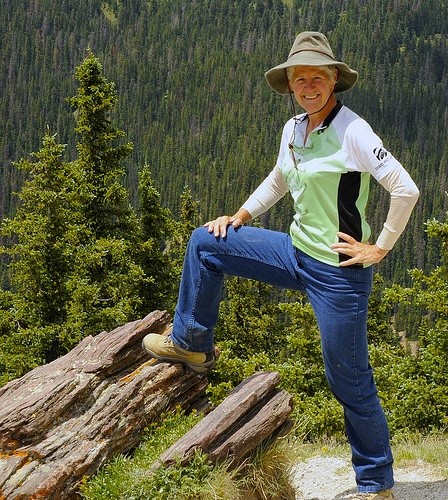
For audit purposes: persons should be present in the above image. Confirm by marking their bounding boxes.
[139,29,420,500]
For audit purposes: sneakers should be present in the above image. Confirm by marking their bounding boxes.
[352,491,394,499]
[142,332,217,374]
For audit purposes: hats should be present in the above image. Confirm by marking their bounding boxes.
[265,31,358,97]
